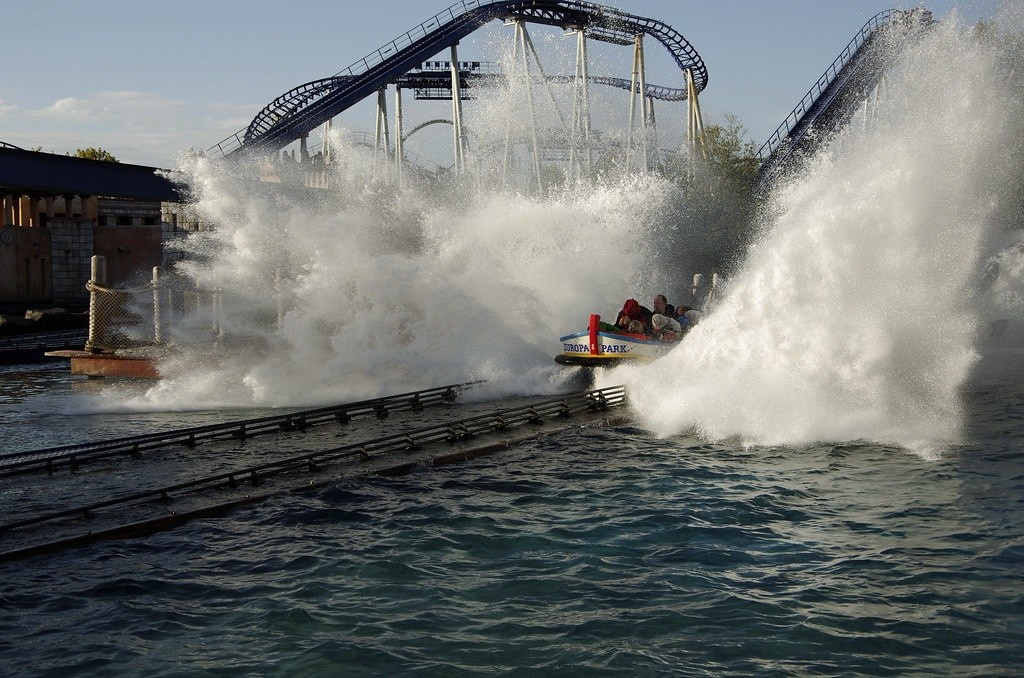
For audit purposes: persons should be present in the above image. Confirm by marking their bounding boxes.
[614,294,700,340]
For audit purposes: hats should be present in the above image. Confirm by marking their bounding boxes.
[623,298,640,318]
[651,314,668,330]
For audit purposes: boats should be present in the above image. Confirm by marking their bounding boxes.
[551,314,688,369]
